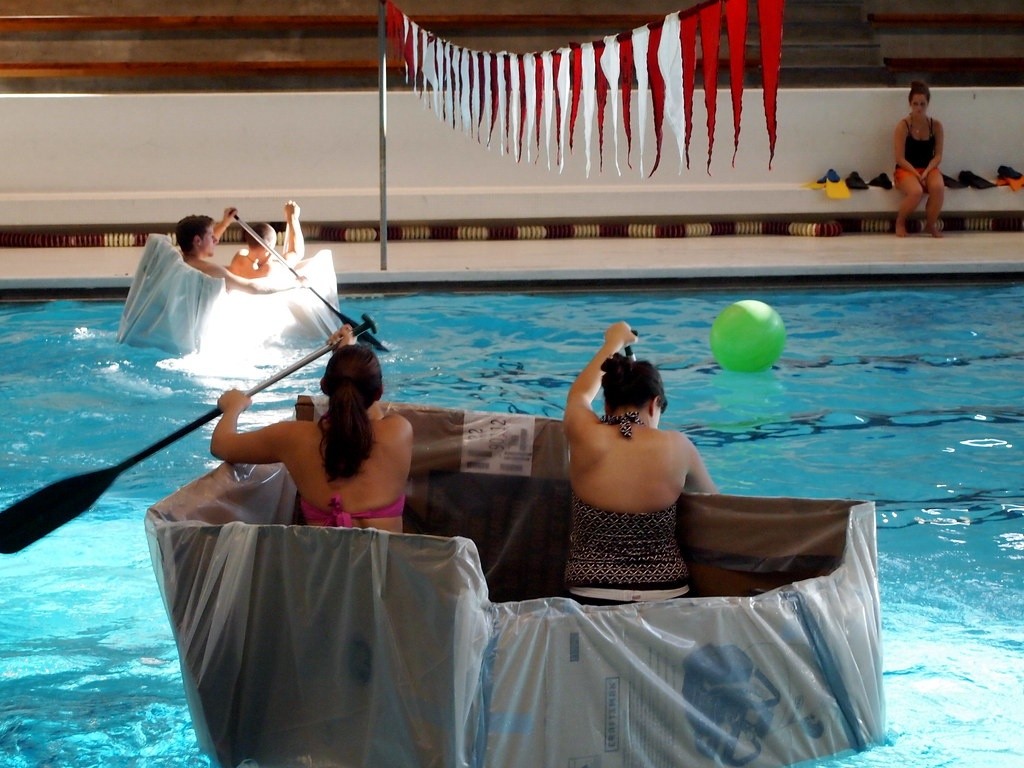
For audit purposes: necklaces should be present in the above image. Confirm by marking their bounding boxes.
[912,124,925,133]
[245,255,271,272]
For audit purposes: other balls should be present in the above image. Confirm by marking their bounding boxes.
[708,299,788,375]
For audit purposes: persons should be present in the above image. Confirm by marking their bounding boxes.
[560,319,717,608]
[176,205,309,296]
[891,81,947,240]
[211,322,412,533]
[229,199,305,279]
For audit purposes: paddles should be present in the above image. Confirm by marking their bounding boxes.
[233,212,393,355]
[1,312,379,556]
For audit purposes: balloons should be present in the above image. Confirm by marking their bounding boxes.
[710,298,788,371]
